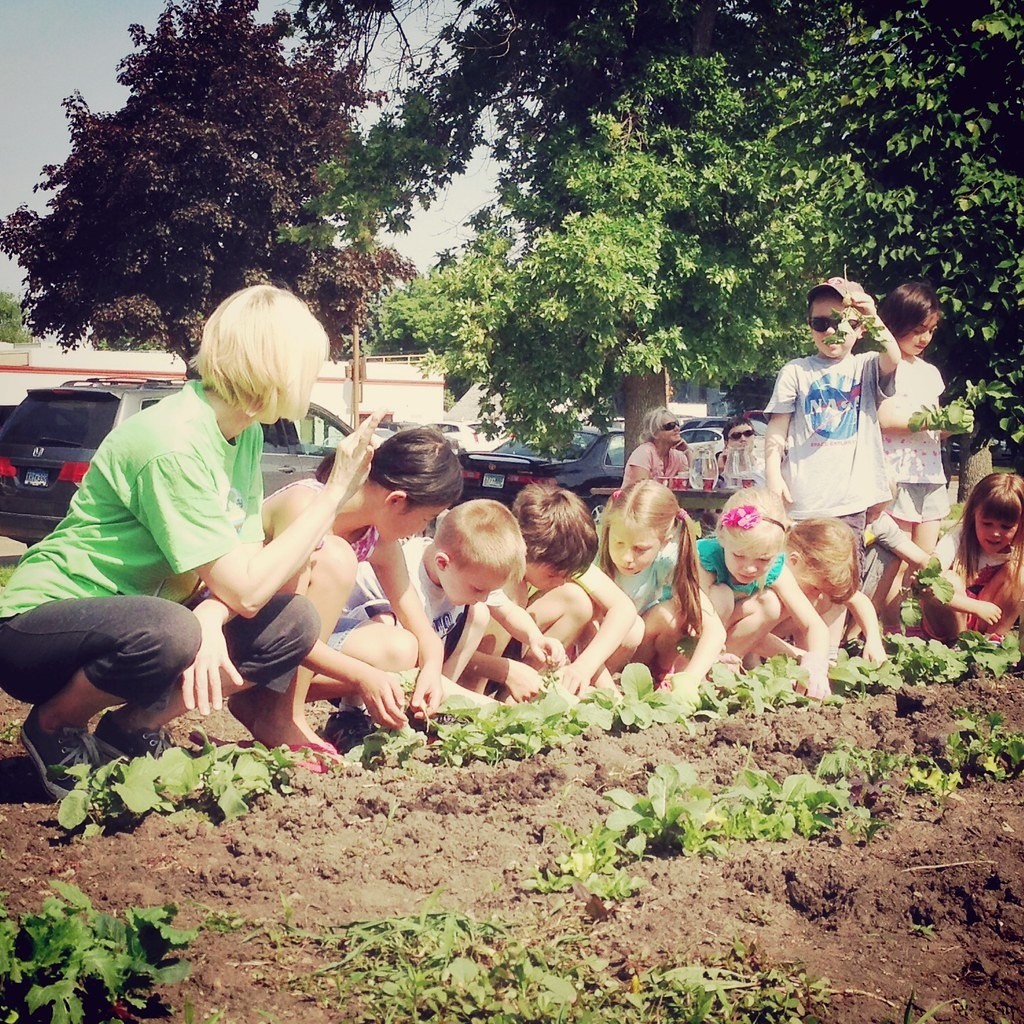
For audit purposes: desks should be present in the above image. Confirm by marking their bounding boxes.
[590,488,737,509]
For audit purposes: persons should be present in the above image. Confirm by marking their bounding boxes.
[0,285,389,803]
[839,474,930,658]
[341,499,527,682]
[741,517,887,669]
[871,283,973,610]
[462,484,637,698]
[695,487,831,702]
[719,416,766,489]
[620,406,692,490]
[764,277,901,669]
[226,429,463,753]
[594,479,727,707]
[921,472,1024,648]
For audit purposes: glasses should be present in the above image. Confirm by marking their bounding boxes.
[653,420,684,436]
[808,314,864,332]
[727,429,755,440]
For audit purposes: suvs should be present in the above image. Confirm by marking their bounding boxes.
[0,375,374,549]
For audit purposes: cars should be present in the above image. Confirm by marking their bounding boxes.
[327,409,772,525]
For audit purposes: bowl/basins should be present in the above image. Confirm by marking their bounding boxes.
[658,477,669,489]
[702,477,715,491]
[742,478,753,487]
[673,477,689,491]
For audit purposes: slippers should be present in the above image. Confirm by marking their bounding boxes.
[264,742,341,772]
[189,729,257,749]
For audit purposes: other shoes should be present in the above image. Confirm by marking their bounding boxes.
[906,625,925,639]
[987,632,1004,646]
[404,707,457,745]
[883,624,901,636]
[324,708,380,755]
[838,638,866,658]
[654,667,673,692]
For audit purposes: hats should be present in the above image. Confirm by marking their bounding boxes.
[805,277,865,306]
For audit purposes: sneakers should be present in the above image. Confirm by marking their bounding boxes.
[92,709,177,766]
[19,705,107,802]
